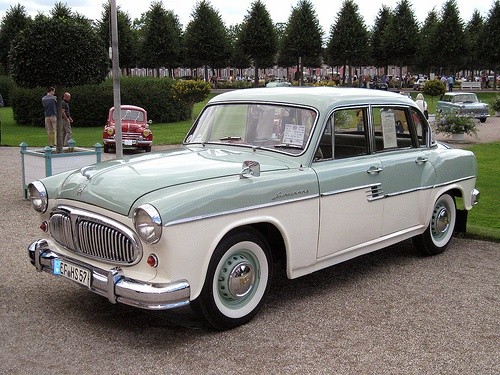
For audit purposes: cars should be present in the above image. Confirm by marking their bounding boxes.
[176,75,196,82]
[453,72,500,89]
[436,91,491,124]
[103,104,153,153]
[218,72,358,88]
[413,78,427,91]
[27,86,481,333]
[366,74,404,90]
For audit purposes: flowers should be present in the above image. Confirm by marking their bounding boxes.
[428,103,483,140]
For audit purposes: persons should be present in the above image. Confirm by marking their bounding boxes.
[42,87,57,149]
[200,71,500,133]
[61,92,74,146]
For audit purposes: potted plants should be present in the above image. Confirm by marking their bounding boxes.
[9,13,104,207]
[451,133,464,144]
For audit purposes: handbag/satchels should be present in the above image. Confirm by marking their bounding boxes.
[424,110,429,120]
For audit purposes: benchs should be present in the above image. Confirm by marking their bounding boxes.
[322,133,423,159]
